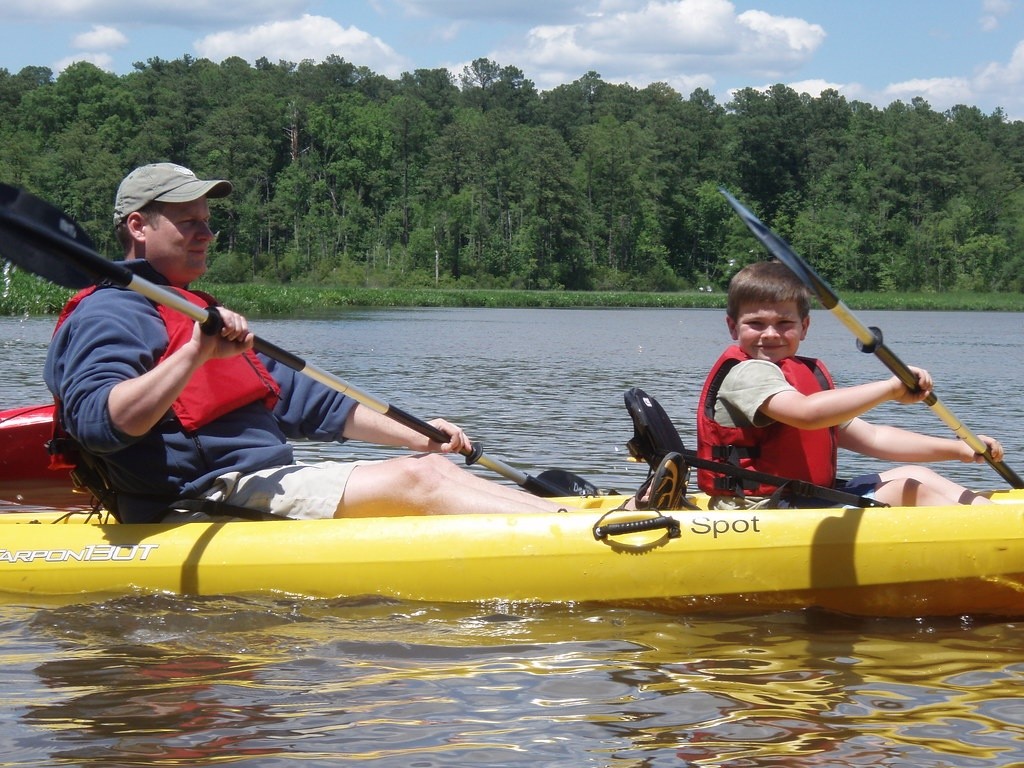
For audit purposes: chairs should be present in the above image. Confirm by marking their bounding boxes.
[623,388,704,510]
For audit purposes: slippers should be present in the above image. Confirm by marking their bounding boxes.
[617,452,688,511]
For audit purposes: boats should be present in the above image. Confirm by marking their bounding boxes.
[0,396,1024,622]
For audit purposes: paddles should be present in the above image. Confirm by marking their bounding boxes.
[716,186,1024,489]
[0,179,602,498]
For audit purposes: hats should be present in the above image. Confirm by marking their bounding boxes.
[113,162,232,228]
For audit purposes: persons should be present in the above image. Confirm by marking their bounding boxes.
[41,166,686,525]
[695,259,1005,505]
